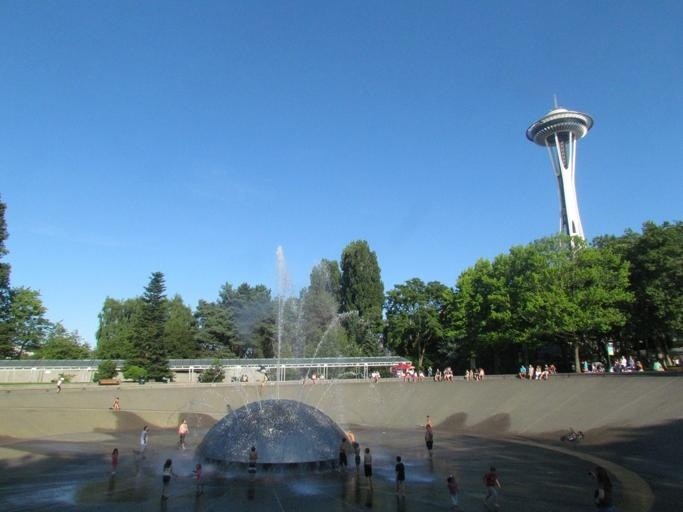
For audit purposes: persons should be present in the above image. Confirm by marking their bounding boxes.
[161,459,179,498]
[111,447,118,475]
[112,397,120,409]
[404,370,425,383]
[139,424,150,454]
[464,367,485,382]
[262,374,269,387]
[56,377,64,393]
[192,464,204,497]
[581,354,683,374]
[519,363,557,381]
[481,466,502,510]
[240,374,249,381]
[340,437,372,485]
[447,473,459,508]
[311,372,325,384]
[427,365,454,383]
[425,415,434,427]
[176,419,190,450]
[371,369,381,384]
[396,455,405,493]
[247,446,258,479]
[425,424,433,457]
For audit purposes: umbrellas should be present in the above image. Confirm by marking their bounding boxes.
[394,363,416,370]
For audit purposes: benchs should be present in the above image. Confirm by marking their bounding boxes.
[99,378,120,385]
